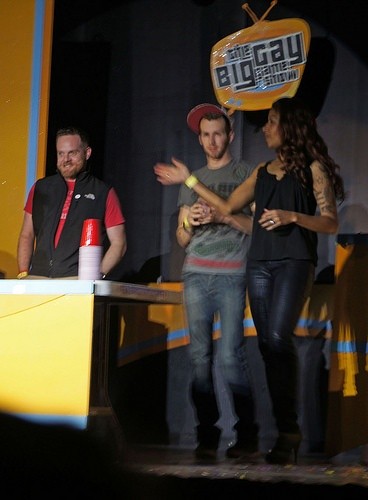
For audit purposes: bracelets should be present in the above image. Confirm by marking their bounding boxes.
[18,271,28,279]
[100,272,107,279]
[181,217,192,234]
[186,175,198,189]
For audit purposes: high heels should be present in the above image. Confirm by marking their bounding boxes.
[263,424,301,466]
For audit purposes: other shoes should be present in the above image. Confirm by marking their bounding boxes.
[194,428,217,461]
[224,429,259,458]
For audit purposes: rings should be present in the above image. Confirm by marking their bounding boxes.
[270,220,275,224]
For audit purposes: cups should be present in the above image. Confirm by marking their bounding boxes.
[78,218,103,280]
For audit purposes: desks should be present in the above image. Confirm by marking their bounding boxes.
[0,280,183,435]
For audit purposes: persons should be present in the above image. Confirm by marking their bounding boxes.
[17,125,127,280]
[171,103,258,456]
[155,98,345,465]
